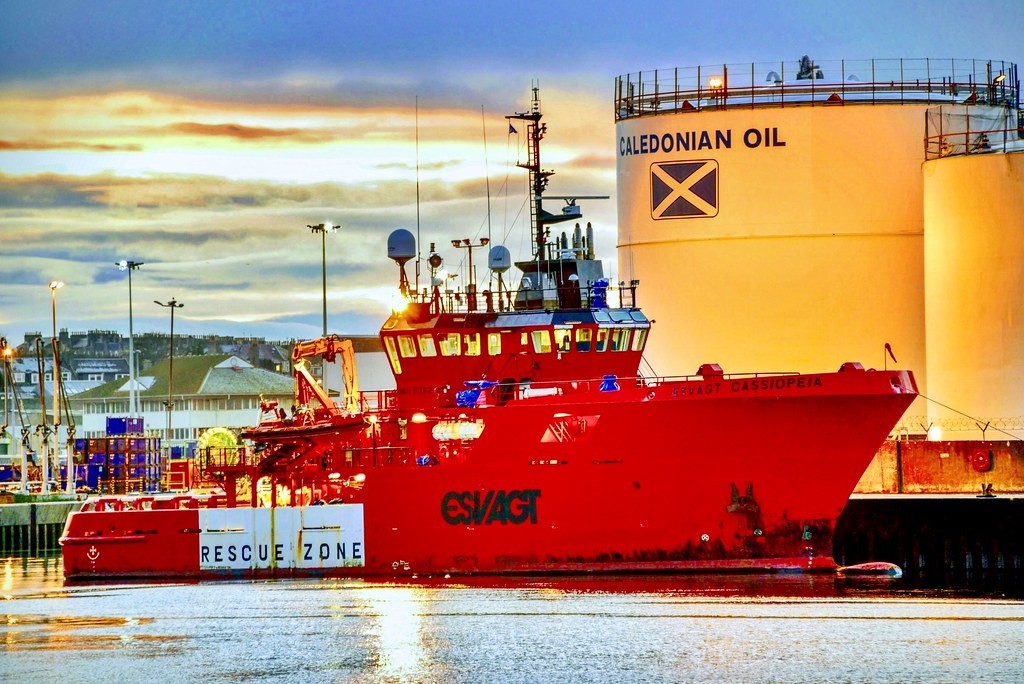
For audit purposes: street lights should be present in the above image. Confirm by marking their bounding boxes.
[2,349,13,431]
[452,238,490,284]
[48,279,64,467]
[114,261,145,417]
[154,297,184,437]
[307,223,342,338]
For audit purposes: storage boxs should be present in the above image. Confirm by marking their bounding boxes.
[1,417,197,494]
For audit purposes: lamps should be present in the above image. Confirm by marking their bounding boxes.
[462,239,470,245]
[618,320,623,323]
[629,280,640,287]
[994,75,1006,84]
[480,238,490,245]
[522,277,533,289]
[452,240,460,246]
[651,320,655,323]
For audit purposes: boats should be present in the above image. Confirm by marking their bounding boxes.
[58,78,918,597]
